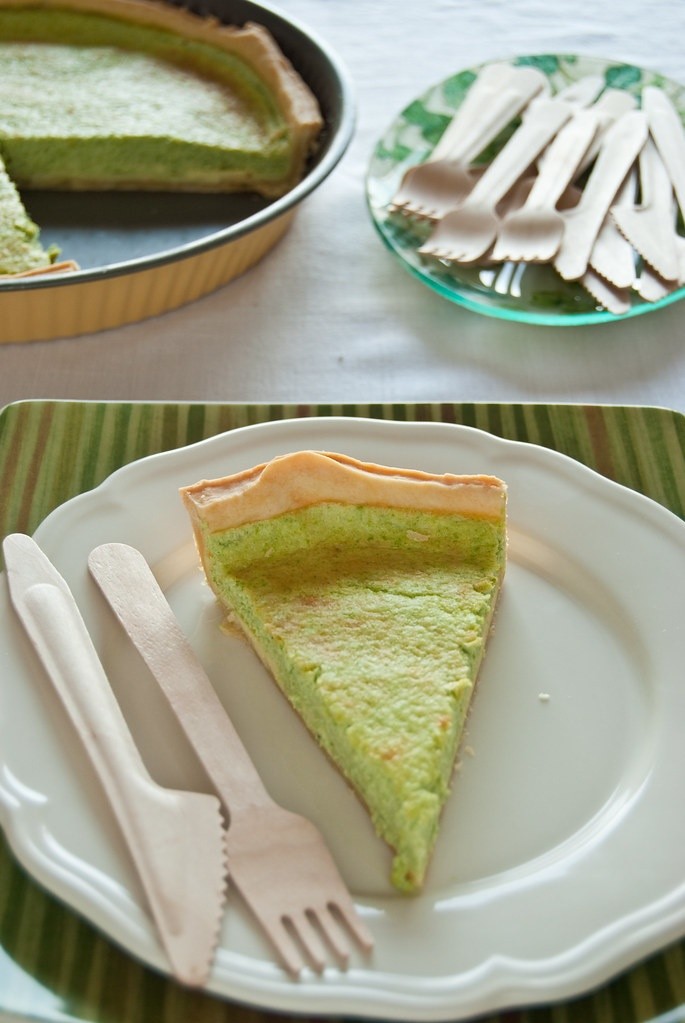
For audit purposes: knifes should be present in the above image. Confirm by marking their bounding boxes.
[388,64,685,317]
[4,532,227,986]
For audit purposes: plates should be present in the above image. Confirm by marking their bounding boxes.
[0,1,356,342]
[366,56,685,325]
[0,416,684,1020]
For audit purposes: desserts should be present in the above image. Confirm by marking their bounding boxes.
[179,450,508,892]
[0,0,325,281]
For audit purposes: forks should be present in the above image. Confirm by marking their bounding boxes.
[86,542,375,976]
